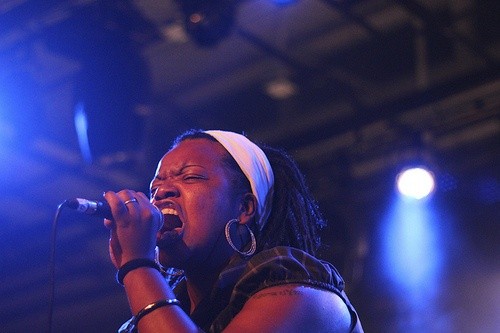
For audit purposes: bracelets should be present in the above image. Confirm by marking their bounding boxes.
[118,259,161,285]
[131,299,181,322]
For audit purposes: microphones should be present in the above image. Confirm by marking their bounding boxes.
[64,197,164,231]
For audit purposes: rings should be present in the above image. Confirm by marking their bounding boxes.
[103,130,364,333]
[124,198,137,204]
